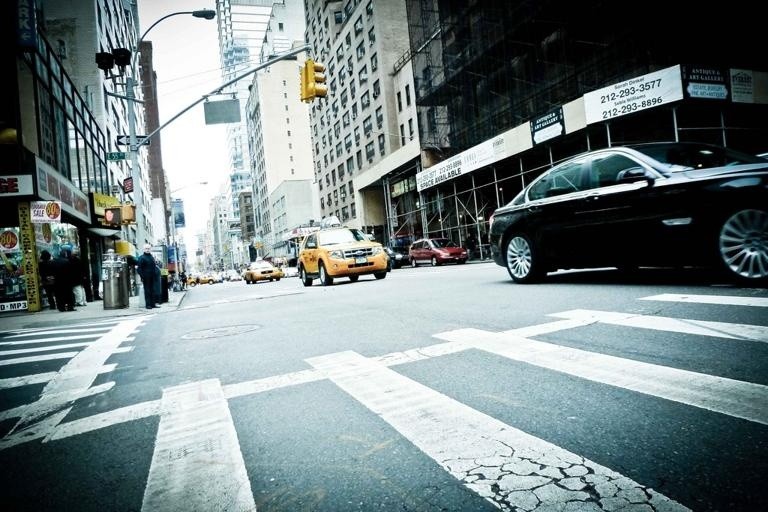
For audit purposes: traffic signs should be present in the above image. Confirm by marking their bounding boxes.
[107,151,129,160]
[116,135,151,147]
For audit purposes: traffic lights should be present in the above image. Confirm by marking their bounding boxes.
[104,208,122,225]
[301,59,328,103]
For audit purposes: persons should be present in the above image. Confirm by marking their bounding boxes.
[137,243,161,309]
[38,250,60,310]
[52,251,78,312]
[167,272,172,291]
[69,246,88,306]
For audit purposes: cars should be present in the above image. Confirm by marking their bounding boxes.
[186,217,468,288]
[489,138,768,286]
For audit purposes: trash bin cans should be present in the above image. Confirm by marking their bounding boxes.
[102,261,129,310]
[160,275,168,302]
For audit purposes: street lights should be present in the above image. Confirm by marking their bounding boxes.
[95,8,215,307]
[171,181,208,290]
